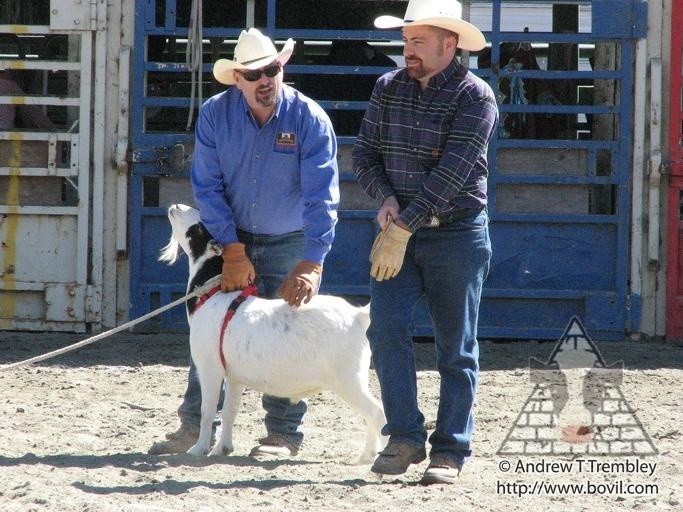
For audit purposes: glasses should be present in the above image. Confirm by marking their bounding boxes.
[234,65,281,81]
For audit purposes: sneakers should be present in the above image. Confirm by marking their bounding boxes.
[421,452,464,485]
[371,436,427,476]
[246,433,299,462]
[146,420,217,458]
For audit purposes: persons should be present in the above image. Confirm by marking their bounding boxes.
[350,0,500,486]
[0,69,57,130]
[149,26,340,456]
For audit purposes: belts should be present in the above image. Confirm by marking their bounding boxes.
[438,205,486,228]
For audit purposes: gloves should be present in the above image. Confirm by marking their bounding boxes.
[368,218,414,283]
[276,260,323,307]
[217,240,257,293]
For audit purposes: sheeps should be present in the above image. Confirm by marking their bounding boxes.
[157,202,388,484]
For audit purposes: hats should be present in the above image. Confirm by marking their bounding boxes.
[371,1,487,51]
[213,27,295,86]
